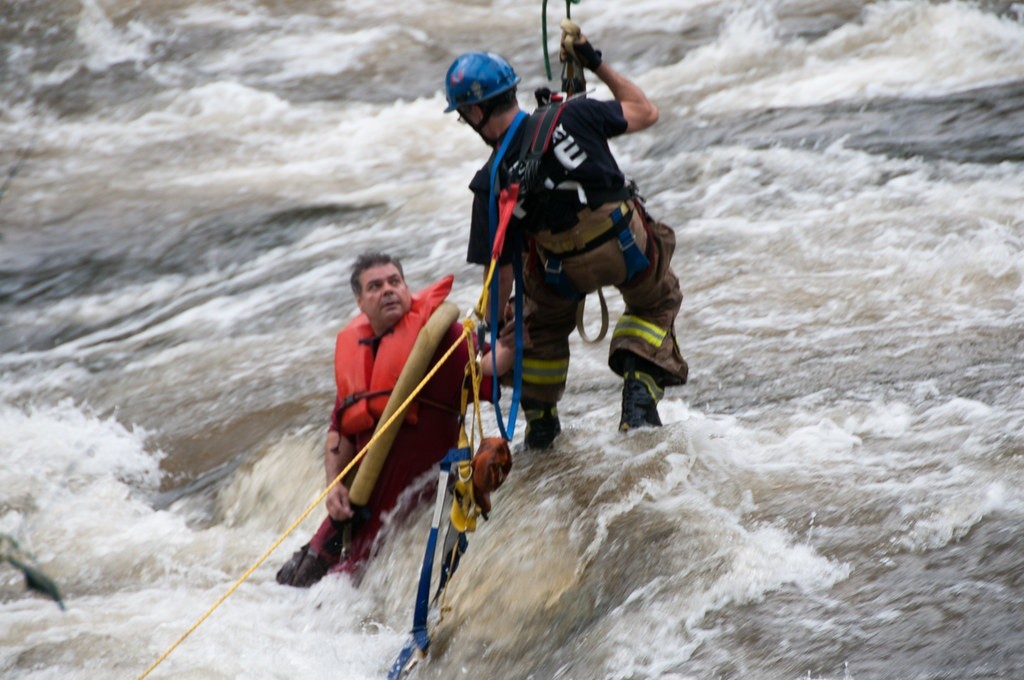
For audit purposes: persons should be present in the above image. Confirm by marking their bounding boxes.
[443,20,687,449]
[276,255,530,587]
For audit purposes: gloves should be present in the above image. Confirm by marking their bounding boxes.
[559,30,601,72]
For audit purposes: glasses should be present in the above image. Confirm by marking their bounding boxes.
[458,108,471,126]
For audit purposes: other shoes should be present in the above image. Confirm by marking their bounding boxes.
[514,418,561,452]
[620,377,662,429]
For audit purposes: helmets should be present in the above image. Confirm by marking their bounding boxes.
[443,52,521,113]
